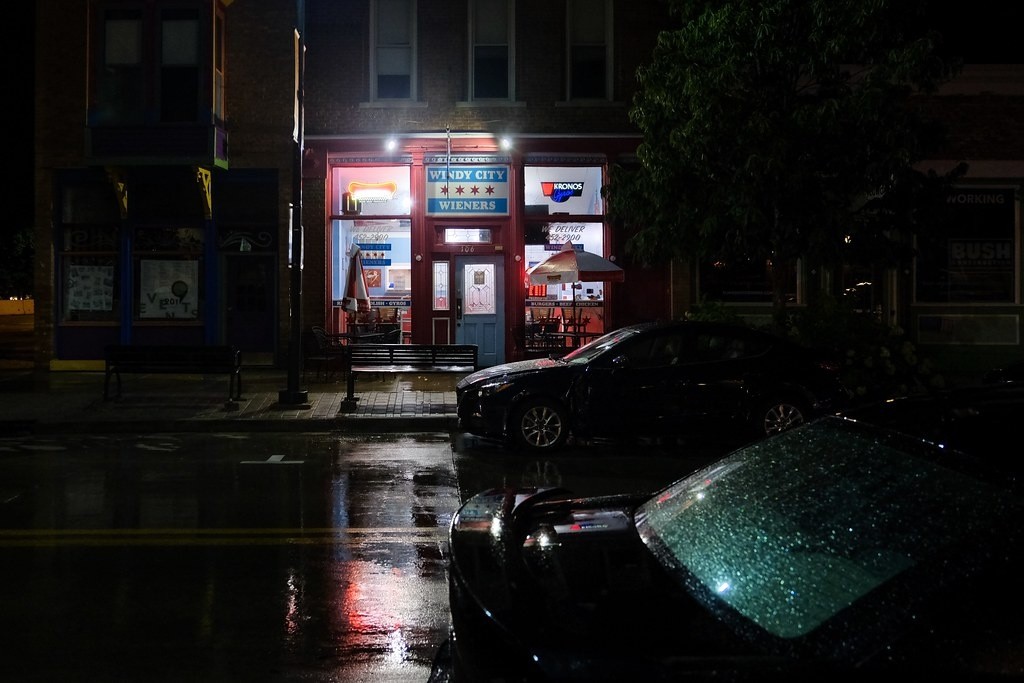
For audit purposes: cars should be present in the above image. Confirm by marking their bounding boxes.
[455,319,896,456]
[445,379,1024,683]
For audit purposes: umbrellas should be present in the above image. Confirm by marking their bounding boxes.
[341,244,372,343]
[526,242,626,332]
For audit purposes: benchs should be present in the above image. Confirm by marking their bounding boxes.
[104,343,243,411]
[345,344,478,405]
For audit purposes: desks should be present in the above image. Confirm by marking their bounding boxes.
[551,332,601,347]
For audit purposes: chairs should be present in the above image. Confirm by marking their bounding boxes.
[532,321,585,348]
[306,326,401,381]
